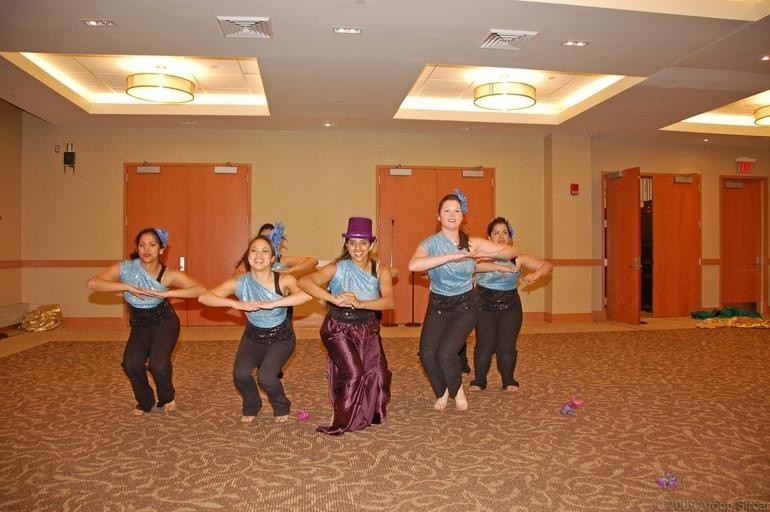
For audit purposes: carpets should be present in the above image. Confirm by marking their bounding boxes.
[0,326,769,512]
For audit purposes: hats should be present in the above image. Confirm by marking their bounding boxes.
[341,217,376,241]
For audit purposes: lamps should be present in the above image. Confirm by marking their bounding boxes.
[753,107,770,128]
[474,82,536,112]
[126,73,195,106]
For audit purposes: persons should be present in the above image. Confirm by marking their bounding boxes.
[232,223,319,379]
[198,236,314,423]
[459,342,471,378]
[296,217,396,435]
[87,227,208,417]
[375,267,399,321]
[468,217,553,392]
[408,194,518,411]
